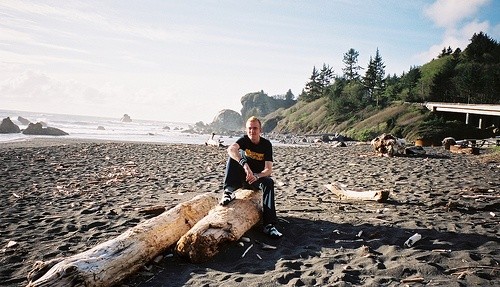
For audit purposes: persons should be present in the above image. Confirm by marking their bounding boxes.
[219,115,282,237]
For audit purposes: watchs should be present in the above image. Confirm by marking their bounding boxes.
[255,174,259,180]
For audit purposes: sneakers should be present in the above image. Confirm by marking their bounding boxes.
[263,223,283,238]
[219,189,236,205]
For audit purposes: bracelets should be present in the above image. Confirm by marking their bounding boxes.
[239,158,247,166]
